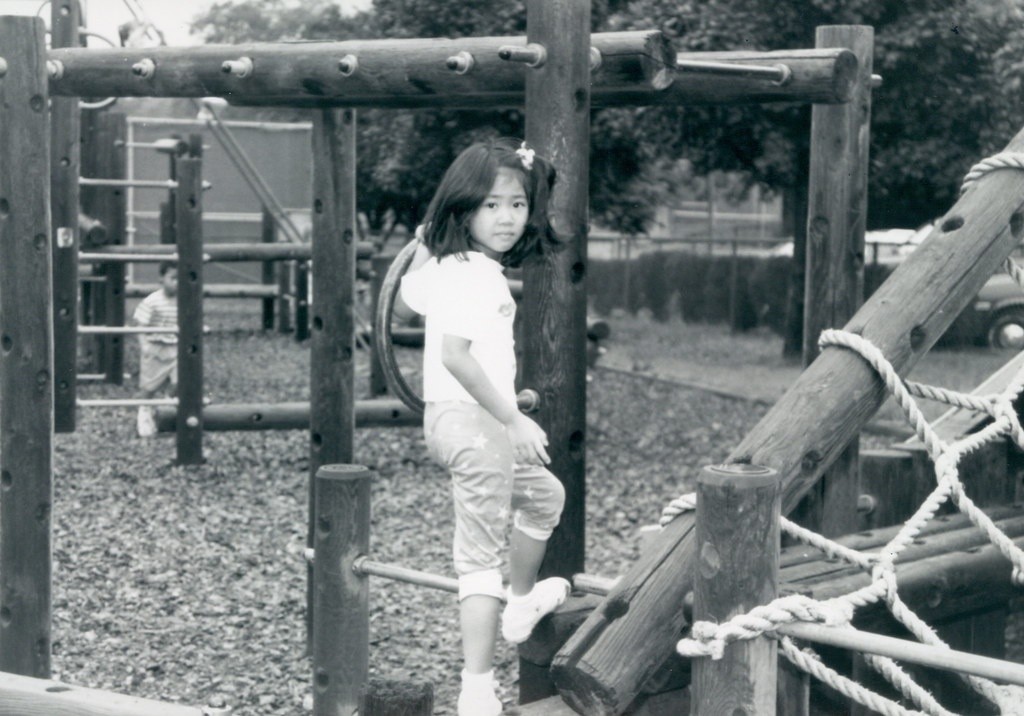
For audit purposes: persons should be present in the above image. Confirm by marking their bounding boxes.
[133,259,179,437]
[400,137,571,716]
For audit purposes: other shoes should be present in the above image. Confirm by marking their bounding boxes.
[137,405,156,439]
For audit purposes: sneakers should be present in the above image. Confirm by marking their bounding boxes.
[458,690,504,716]
[501,577,570,643]
